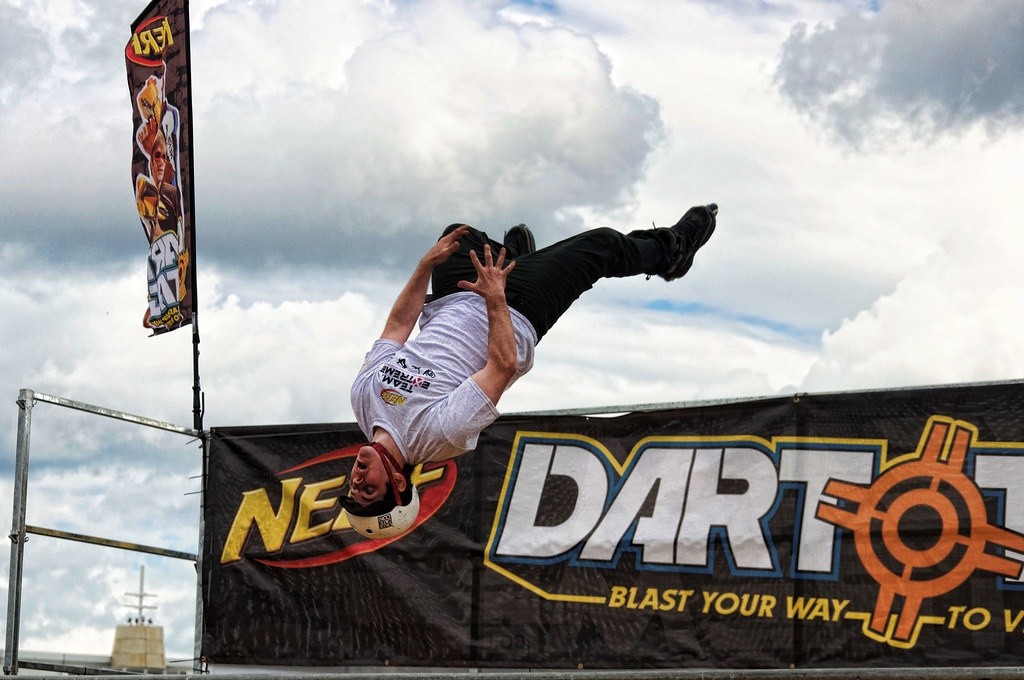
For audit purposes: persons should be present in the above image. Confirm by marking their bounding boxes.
[337,204,717,540]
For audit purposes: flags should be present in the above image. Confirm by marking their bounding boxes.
[123,0,198,339]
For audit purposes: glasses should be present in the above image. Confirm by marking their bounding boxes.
[152,151,165,161]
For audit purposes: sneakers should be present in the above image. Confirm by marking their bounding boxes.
[504,223,536,258]
[653,205,716,282]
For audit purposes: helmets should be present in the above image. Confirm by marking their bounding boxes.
[338,484,420,539]
